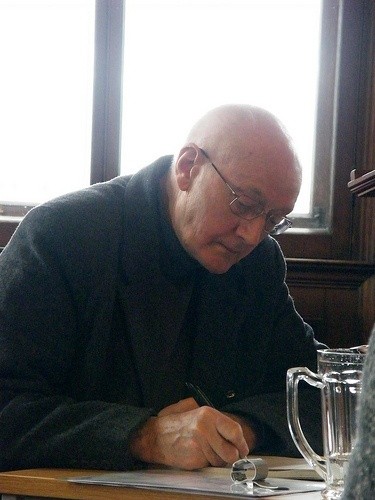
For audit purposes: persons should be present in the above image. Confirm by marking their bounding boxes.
[0,105,356,471]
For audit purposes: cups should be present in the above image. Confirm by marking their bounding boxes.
[286,348,368,499]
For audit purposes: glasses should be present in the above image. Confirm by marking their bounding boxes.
[199,148,292,235]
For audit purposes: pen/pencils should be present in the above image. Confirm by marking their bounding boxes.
[182,380,213,408]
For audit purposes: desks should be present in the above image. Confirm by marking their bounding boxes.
[0,454,333,500]
